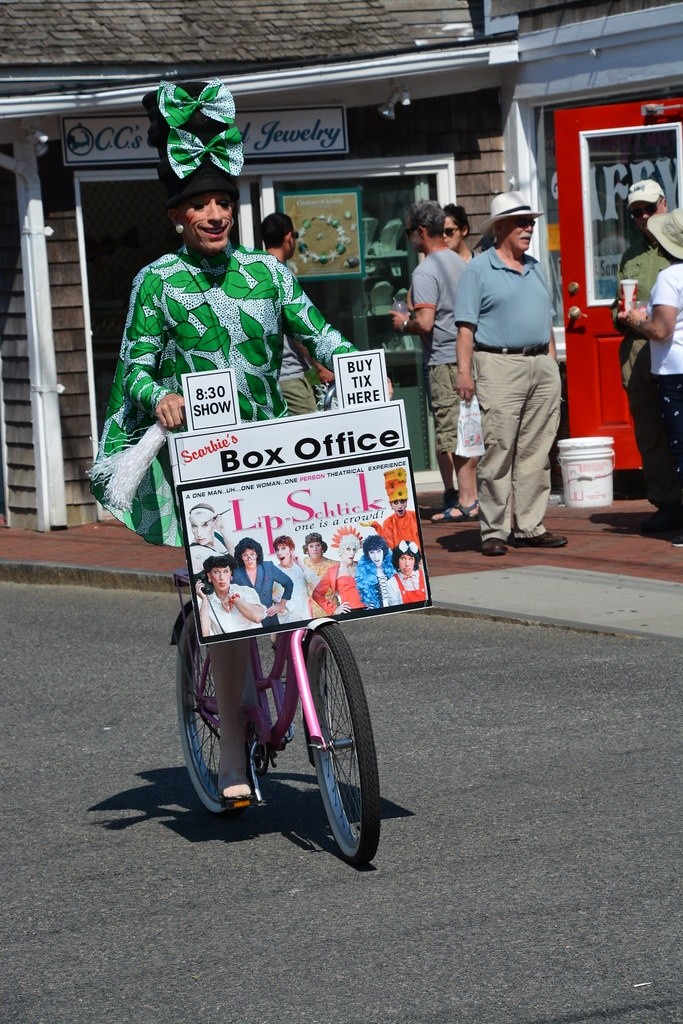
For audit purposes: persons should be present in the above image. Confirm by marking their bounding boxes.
[260,212,333,414]
[190,468,427,637]
[88,82,392,808]
[456,193,568,553]
[389,201,481,523]
[611,179,683,546]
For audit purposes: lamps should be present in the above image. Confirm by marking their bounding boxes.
[30,127,49,144]
[378,87,403,120]
[402,84,411,105]
[26,134,48,157]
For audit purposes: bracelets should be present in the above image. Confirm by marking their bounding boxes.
[401,320,409,332]
[229,593,240,606]
[637,320,646,329]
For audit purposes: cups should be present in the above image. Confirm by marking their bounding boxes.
[392,301,409,332]
[620,279,638,314]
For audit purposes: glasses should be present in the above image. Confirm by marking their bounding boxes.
[631,199,662,217]
[500,218,535,227]
[443,227,463,236]
[406,224,427,237]
[240,553,258,561]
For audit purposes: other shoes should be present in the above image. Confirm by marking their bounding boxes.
[216,760,251,799]
[443,490,459,509]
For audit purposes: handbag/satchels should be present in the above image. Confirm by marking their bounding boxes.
[455,395,485,457]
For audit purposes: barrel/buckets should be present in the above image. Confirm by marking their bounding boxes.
[557,436,616,508]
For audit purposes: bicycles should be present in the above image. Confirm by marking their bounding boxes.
[171,407,381,865]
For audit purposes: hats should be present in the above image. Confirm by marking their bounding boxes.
[648,208,683,260]
[626,180,665,210]
[142,77,243,208]
[479,191,544,236]
[303,533,328,554]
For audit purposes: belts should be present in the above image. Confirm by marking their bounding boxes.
[474,343,548,355]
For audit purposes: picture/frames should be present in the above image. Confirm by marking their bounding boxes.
[278,187,367,282]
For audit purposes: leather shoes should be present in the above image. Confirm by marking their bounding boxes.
[513,532,567,548]
[482,538,506,554]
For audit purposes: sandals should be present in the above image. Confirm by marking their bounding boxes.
[431,502,478,522]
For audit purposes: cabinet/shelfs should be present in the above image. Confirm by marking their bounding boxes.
[347,170,428,471]
